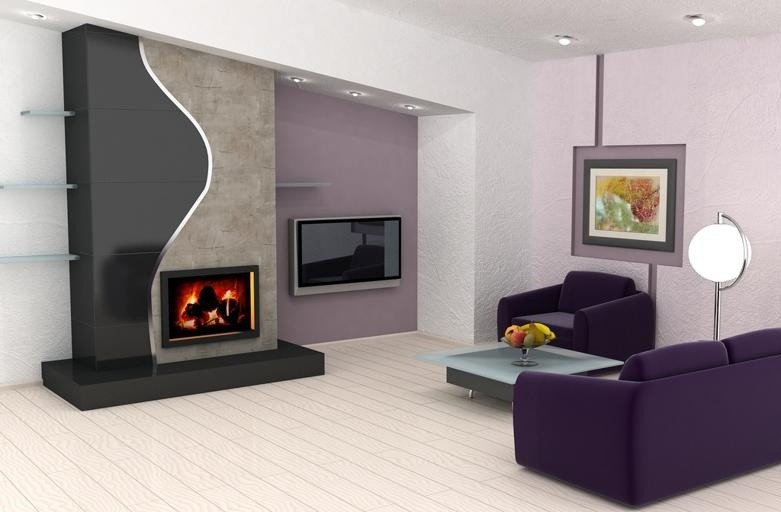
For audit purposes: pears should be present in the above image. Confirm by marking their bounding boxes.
[528,320,545,347]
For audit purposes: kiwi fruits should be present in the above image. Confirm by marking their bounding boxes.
[522,333,536,346]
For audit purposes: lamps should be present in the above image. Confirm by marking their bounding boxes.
[688,212,752,342]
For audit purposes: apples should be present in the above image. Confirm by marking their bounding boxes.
[510,327,523,345]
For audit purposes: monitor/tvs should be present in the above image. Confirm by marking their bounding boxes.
[288,215,404,294]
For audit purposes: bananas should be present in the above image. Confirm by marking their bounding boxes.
[523,322,557,341]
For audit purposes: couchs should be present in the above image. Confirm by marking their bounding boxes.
[496,270,654,377]
[514,328,781,508]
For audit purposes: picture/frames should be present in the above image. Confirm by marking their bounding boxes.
[582,158,677,252]
